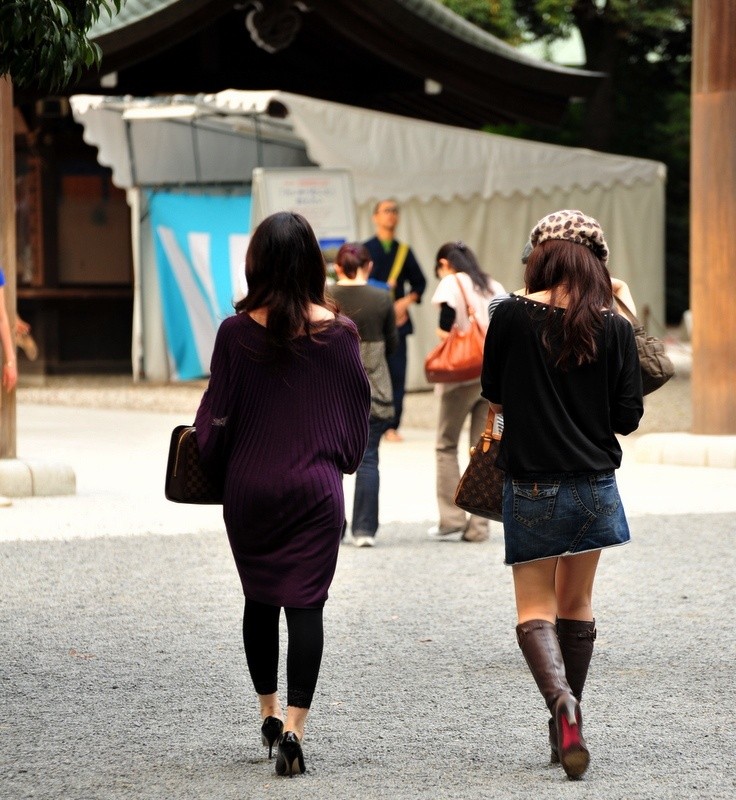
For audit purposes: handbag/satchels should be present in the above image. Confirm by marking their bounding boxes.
[423,274,486,381]
[612,292,674,396]
[164,424,222,505]
[454,408,504,521]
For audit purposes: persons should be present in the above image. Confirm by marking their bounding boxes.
[426,242,510,542]
[0,266,37,392]
[323,242,405,546]
[360,198,428,441]
[480,208,644,781]
[488,239,638,439]
[195,213,372,779]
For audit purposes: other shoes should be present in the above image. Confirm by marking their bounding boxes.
[429,523,463,537]
[354,535,374,547]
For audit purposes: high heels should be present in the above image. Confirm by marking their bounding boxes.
[275,731,305,777]
[260,716,284,759]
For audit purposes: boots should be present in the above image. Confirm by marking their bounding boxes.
[516,619,589,779]
[548,616,597,761]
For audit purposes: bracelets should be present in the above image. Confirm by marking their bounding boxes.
[5,359,16,368]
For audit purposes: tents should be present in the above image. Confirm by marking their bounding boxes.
[67,84,669,390]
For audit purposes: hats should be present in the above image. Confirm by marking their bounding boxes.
[528,209,608,264]
